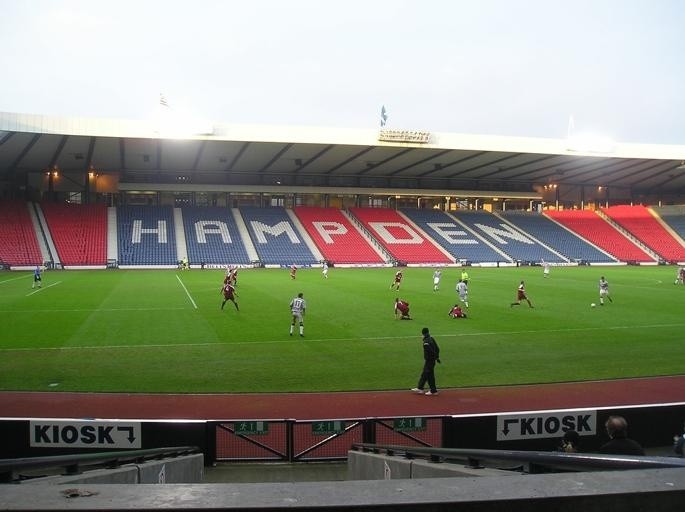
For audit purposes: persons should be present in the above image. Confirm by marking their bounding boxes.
[447,304,467,319]
[393,297,412,321]
[287,292,307,337]
[540,258,551,278]
[389,270,403,292]
[290,262,297,279]
[322,262,329,279]
[455,278,469,308]
[673,262,685,286]
[595,414,647,472]
[554,430,585,453]
[596,276,613,306]
[432,268,442,293]
[30,263,44,290]
[509,279,536,310]
[217,266,242,314]
[409,327,441,396]
[460,268,469,286]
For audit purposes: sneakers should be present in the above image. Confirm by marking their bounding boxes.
[424,390,438,395]
[411,388,425,393]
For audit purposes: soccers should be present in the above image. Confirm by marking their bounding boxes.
[591,303,596,308]
